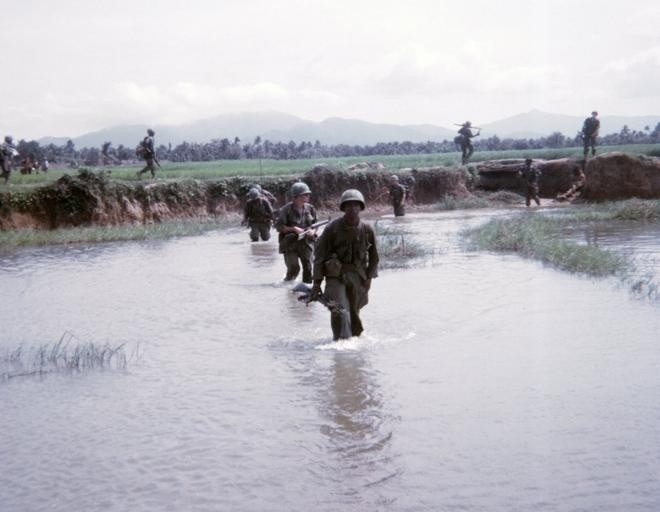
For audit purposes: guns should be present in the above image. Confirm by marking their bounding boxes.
[284,219,329,246]
[292,282,347,318]
[141,142,164,172]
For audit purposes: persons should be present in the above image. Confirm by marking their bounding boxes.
[458,119,480,166]
[581,110,601,158]
[386,175,409,217]
[0,134,49,186]
[307,188,380,342]
[240,187,274,243]
[554,164,585,202]
[244,183,278,233]
[134,128,161,181]
[273,180,319,284]
[518,158,545,207]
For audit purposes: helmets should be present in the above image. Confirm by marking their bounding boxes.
[339,189,366,212]
[250,184,262,199]
[291,182,312,196]
